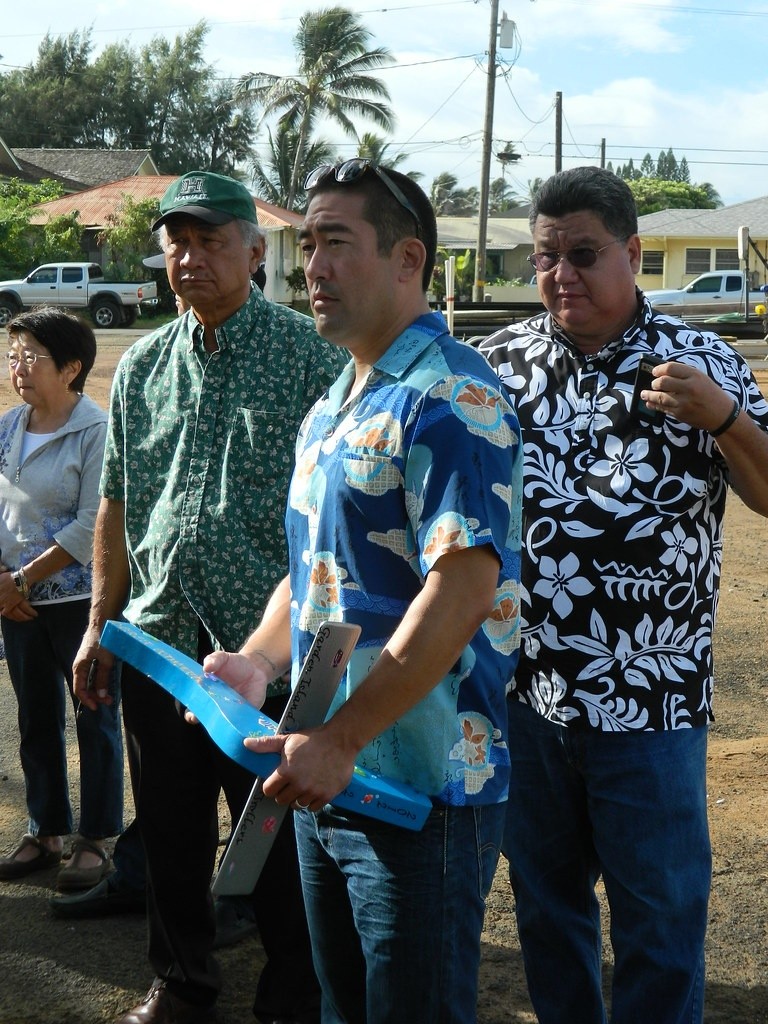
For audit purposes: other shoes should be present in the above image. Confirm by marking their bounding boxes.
[56,839,108,893]
[0,837,61,881]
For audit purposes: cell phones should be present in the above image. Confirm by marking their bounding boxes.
[630,354,671,428]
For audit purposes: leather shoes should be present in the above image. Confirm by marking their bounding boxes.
[116,977,224,1024]
[51,876,150,919]
[213,897,256,949]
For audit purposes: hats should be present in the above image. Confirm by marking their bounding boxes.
[152,170,258,232]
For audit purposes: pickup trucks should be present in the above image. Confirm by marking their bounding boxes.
[643,270,768,321]
[0,262,162,329]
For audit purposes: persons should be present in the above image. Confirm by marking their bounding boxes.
[479,165,768,1024]
[0,310,129,913]
[183,160,525,1024]
[71,174,353,1024]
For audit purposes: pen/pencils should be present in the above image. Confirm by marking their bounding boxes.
[75,658,98,723]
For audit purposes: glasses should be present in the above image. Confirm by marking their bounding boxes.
[304,158,422,224]
[5,350,54,367]
[527,239,629,272]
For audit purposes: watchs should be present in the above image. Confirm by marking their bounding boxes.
[12,571,23,592]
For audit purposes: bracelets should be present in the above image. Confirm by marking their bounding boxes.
[19,568,29,599]
[710,399,740,437]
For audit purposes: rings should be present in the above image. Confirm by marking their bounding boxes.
[295,800,309,810]
